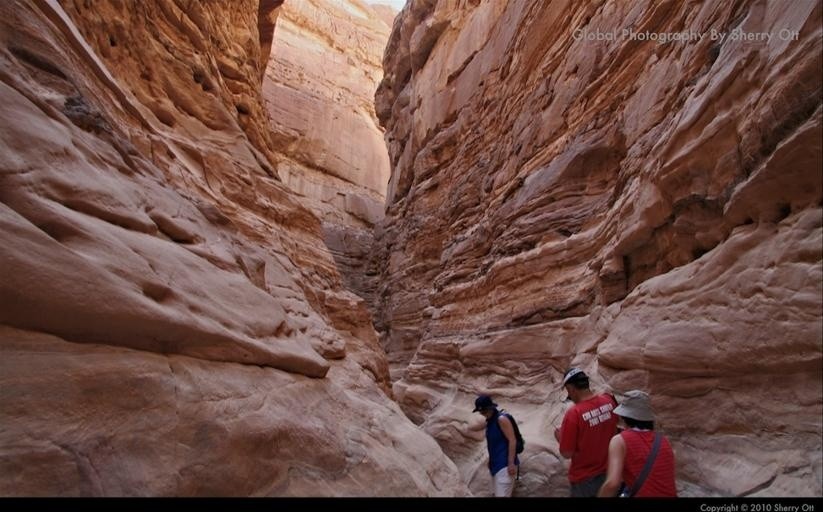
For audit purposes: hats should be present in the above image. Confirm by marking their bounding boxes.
[472,395,498,413]
[613,389,658,422]
[560,367,584,390]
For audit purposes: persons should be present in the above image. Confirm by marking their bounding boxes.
[471,393,520,497]
[595,389,677,497]
[553,367,618,496]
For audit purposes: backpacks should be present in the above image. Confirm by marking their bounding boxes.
[504,412,524,454]
